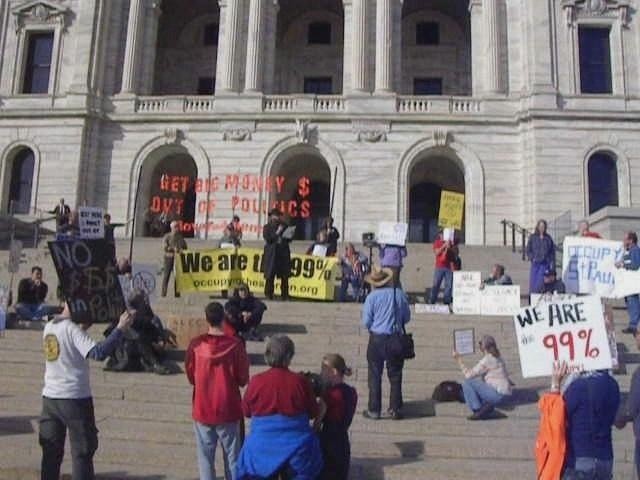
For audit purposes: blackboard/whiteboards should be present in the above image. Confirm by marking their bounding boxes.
[47,238,127,323]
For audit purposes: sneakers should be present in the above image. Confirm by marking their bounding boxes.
[467,404,495,419]
[621,326,637,334]
[362,410,380,420]
[387,407,400,419]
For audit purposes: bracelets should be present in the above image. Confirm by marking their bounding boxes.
[550,384,562,390]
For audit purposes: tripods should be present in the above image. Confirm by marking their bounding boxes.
[357,247,372,302]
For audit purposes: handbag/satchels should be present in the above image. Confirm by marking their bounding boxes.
[384,332,416,360]
[431,382,462,402]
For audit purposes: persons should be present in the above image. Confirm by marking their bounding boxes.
[225,284,266,341]
[114,257,133,280]
[160,220,187,298]
[614,232,640,333]
[13,265,50,322]
[362,263,411,420]
[451,335,513,420]
[428,227,458,305]
[37,293,133,480]
[534,358,619,480]
[263,208,295,301]
[306,216,407,301]
[218,215,243,298]
[238,336,322,478]
[613,323,640,480]
[48,198,71,225]
[104,213,134,264]
[527,219,603,296]
[480,263,512,290]
[104,307,178,376]
[314,354,358,479]
[186,302,249,479]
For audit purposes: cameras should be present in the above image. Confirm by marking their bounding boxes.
[362,232,376,247]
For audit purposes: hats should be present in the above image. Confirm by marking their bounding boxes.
[479,335,495,346]
[543,269,557,276]
[363,266,393,287]
[268,208,283,216]
